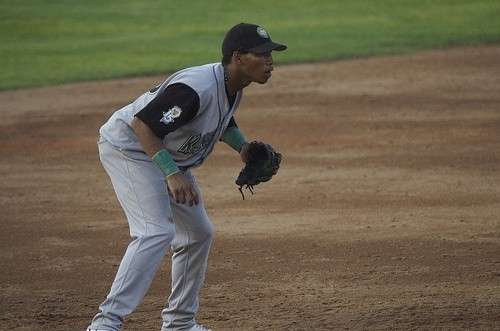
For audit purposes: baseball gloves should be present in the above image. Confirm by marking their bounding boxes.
[236,140,282,201]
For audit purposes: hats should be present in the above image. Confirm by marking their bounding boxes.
[222,22,287,54]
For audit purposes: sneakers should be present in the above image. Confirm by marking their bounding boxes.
[162,323,209,331]
[86,327,110,331]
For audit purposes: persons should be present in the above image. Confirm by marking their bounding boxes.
[87,22,289,331]
